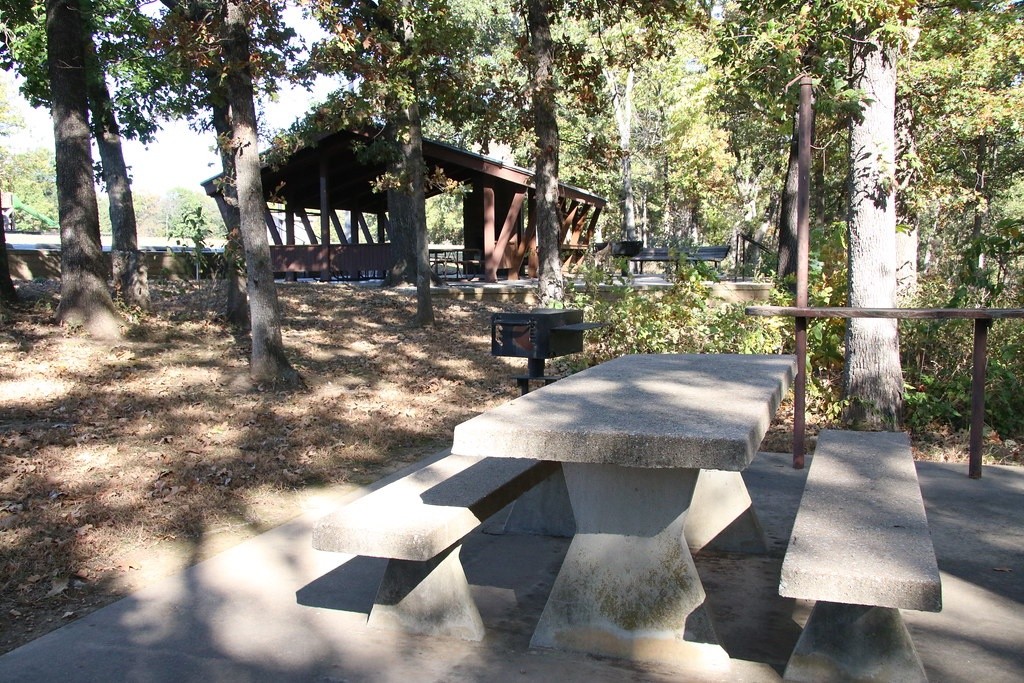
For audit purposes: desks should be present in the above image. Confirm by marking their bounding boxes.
[452,354,799,669]
[428,249,481,278]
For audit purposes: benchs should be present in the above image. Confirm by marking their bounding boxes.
[311,454,579,642]
[429,258,485,281]
[778,427,943,682]
[628,246,731,275]
